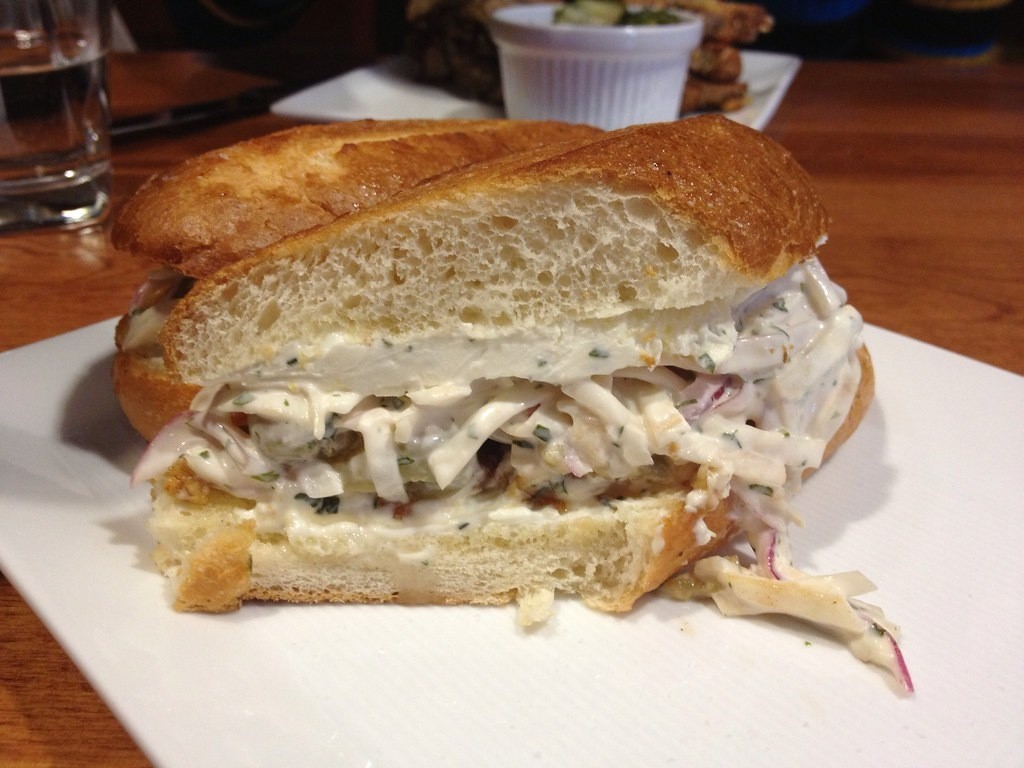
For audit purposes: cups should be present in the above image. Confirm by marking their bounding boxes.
[0,0,110,234]
[487,4,705,144]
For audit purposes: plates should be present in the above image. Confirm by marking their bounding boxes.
[2,178,1023,768]
[271,35,802,165]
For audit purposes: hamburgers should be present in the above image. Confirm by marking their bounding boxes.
[107,117,610,451]
[136,113,875,616]
